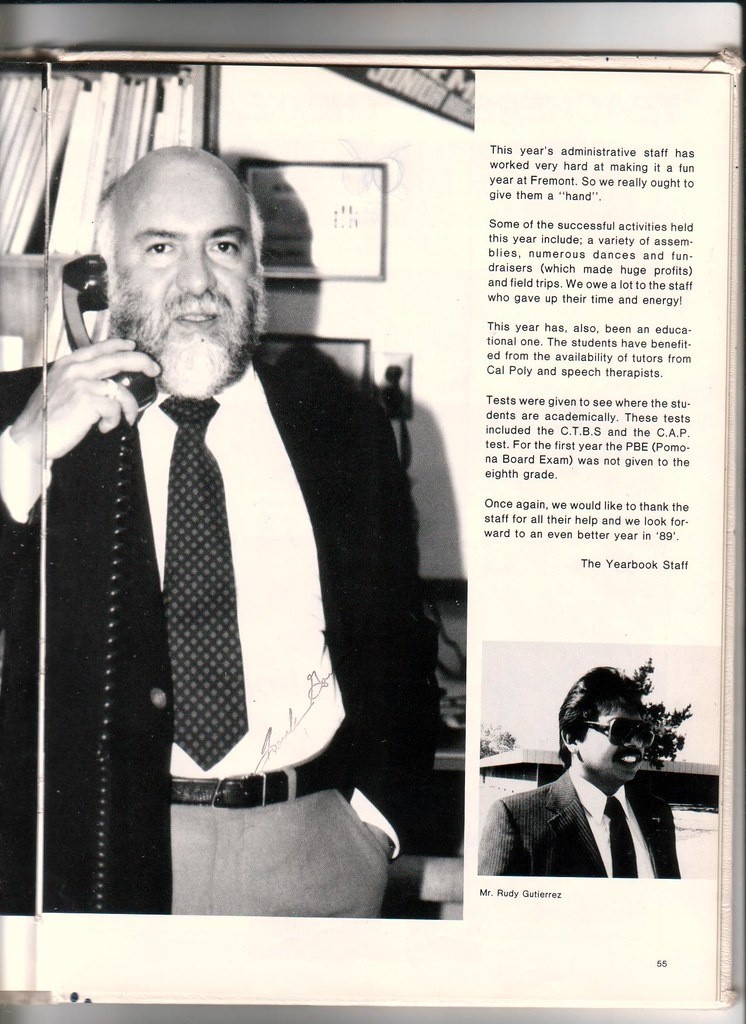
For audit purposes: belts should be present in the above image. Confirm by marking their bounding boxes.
[165,759,354,811]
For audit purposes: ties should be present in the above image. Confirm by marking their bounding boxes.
[159,392,261,766]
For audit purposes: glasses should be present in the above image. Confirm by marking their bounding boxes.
[583,719,673,755]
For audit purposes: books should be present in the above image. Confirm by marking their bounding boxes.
[0,71,193,255]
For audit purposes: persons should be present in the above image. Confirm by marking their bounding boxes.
[477,666,682,880]
[0,147,419,919]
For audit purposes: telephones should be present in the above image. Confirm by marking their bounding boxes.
[60,252,160,421]
